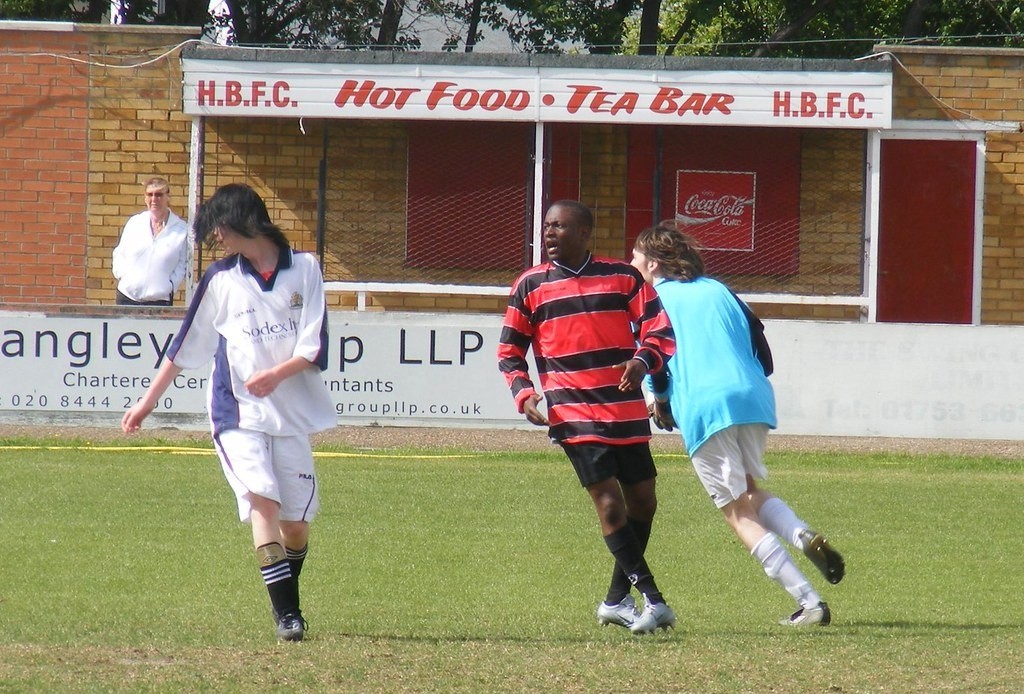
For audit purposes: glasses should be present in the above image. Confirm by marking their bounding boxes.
[145,192,167,197]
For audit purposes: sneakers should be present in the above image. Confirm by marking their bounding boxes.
[631,592,675,634]
[778,601,831,627]
[272,608,281,626]
[798,529,845,585]
[279,612,309,641]
[597,592,642,627]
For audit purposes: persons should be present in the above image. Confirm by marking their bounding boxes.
[122,184,336,640]
[499,201,676,635]
[630,223,845,625]
[112,177,187,306]
